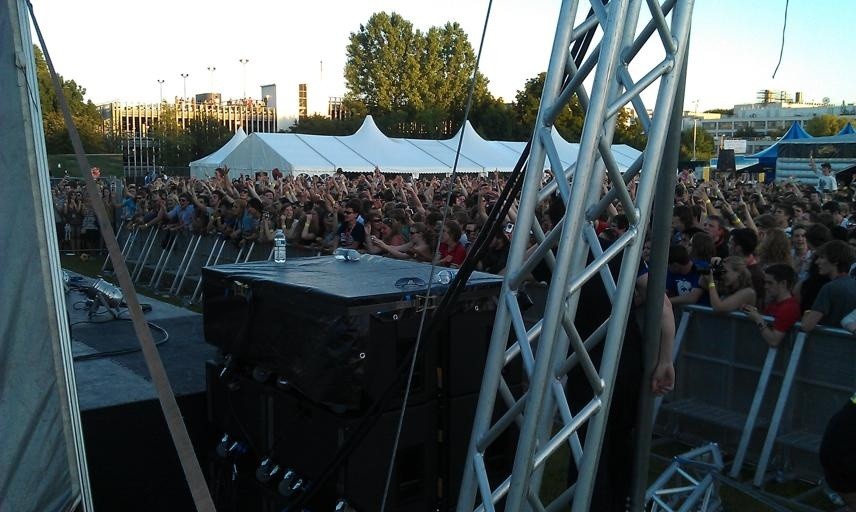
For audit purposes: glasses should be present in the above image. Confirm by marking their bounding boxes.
[344,211,353,215]
[179,199,185,202]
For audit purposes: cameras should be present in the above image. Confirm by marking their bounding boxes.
[707,260,726,281]
[371,236,377,241]
[263,211,270,218]
[505,223,514,233]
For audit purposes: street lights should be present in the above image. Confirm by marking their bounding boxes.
[238,58,249,97]
[156,77,165,103]
[206,65,217,101]
[692,117,699,158]
[179,72,189,129]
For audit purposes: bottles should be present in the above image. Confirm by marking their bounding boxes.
[333,248,361,262]
[821,481,845,505]
[434,268,471,283]
[274,229,286,263]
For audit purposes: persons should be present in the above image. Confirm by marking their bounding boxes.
[820,392,856,512]
[497,194,676,512]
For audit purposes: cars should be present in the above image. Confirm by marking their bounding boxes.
[49,176,146,194]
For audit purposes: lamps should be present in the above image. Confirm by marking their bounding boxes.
[85,275,134,316]
